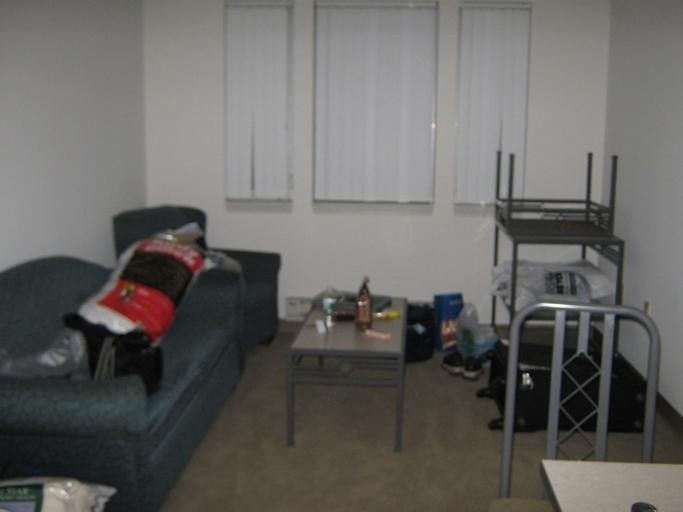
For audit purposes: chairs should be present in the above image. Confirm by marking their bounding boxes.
[110,202,281,357]
[484,301,662,512]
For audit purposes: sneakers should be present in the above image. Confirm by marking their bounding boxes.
[463,357,482,379]
[442,351,464,375]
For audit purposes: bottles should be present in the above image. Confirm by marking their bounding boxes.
[354,275,374,333]
[322,284,344,316]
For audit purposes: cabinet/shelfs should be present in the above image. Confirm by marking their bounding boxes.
[482,149,625,350]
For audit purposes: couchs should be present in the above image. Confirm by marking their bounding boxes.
[1,254,244,512]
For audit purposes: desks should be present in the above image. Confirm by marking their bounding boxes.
[535,460,683,512]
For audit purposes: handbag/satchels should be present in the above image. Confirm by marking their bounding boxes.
[86,324,163,393]
[406,306,434,361]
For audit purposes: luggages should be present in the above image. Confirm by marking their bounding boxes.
[477,340,646,433]
